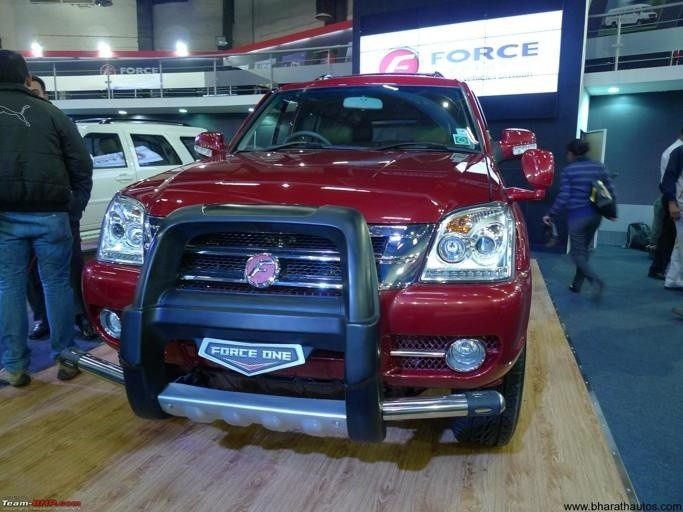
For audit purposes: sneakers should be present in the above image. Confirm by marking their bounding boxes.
[56,356,80,378]
[648,268,666,280]
[664,285,682,292]
[569,284,578,292]
[589,279,603,302]
[673,307,682,317]
[1,368,32,387]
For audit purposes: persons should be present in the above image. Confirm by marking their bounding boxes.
[23,73,103,341]
[540,138,621,299]
[661,143,682,289]
[0,47,92,387]
[648,133,682,281]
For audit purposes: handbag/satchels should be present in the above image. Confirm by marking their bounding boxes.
[591,178,614,215]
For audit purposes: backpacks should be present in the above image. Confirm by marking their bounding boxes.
[626,223,651,250]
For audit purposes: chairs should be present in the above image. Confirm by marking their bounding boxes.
[311,116,452,149]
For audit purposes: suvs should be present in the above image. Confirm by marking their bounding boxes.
[70,116,212,245]
[58,73,555,449]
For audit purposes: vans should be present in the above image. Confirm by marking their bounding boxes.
[604,4,657,29]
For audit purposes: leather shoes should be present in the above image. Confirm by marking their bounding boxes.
[79,319,99,340]
[31,320,48,340]
